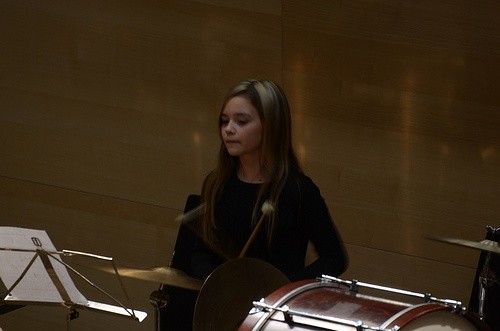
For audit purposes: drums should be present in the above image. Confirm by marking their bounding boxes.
[236,274,494,331]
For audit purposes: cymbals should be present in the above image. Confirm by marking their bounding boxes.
[422,235,500,255]
[193,258,291,331]
[100,265,204,290]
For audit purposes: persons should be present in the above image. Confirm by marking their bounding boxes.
[192,78,351,284]
